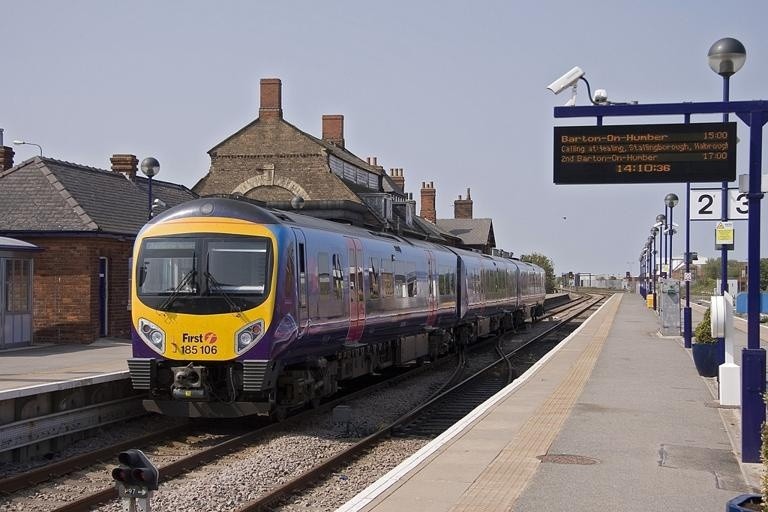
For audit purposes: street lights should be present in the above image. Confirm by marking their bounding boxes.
[141,156,161,221]
[636,192,679,312]
[707,35,748,385]
[14,140,42,159]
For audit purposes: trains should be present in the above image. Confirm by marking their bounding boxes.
[124,191,550,441]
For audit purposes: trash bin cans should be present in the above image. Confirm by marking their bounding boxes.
[646,293,654,308]
